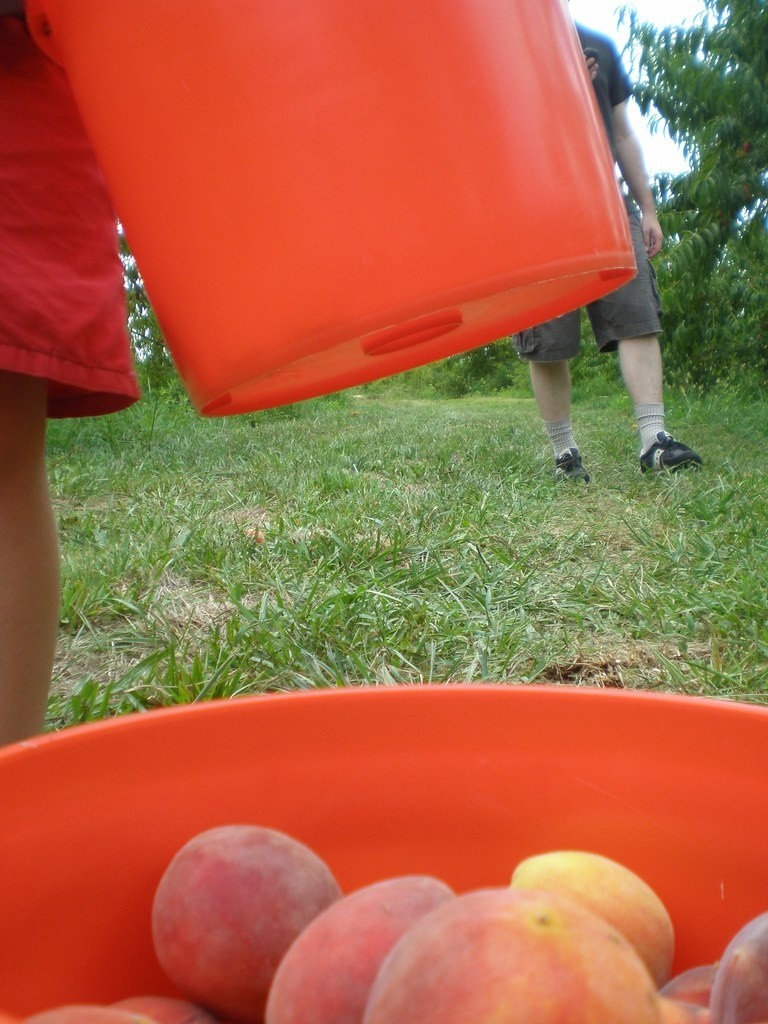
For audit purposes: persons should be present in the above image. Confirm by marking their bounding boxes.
[514,21,705,484]
[0,0,140,747]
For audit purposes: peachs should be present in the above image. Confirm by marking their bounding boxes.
[20,823,768,1024]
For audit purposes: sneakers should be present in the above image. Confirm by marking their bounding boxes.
[639,430,704,477]
[555,447,591,484]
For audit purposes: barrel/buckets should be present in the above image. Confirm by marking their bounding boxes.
[0,686,768,1024]
[20,0,638,416]
